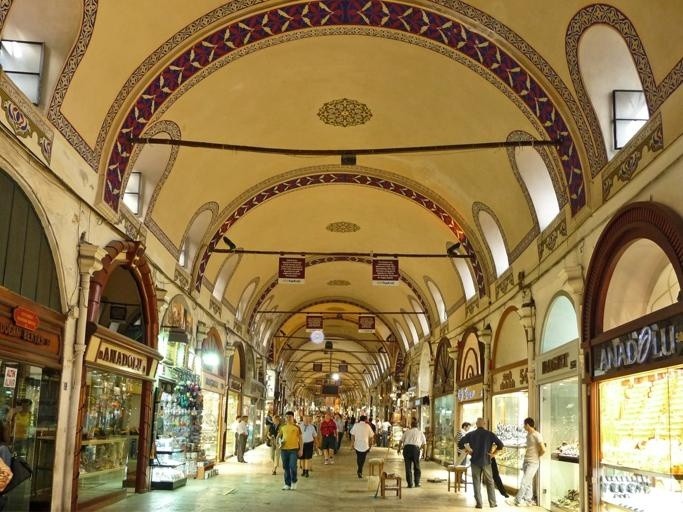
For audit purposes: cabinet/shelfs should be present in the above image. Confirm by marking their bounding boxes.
[71,437,133,505]
[597,460,682,512]
[149,438,187,489]
[548,449,579,512]
[491,440,529,498]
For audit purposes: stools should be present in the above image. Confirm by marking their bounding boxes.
[446,464,468,493]
[366,457,384,490]
[379,471,401,501]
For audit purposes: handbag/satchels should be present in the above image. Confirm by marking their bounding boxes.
[266,438,273,447]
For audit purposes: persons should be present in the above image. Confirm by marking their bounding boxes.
[396,421,428,488]
[260,409,454,478]
[504,416,545,506]
[236,414,248,463]
[457,417,504,509]
[274,410,303,490]
[453,421,470,484]
[231,415,240,456]
[491,457,509,497]
[0,398,32,512]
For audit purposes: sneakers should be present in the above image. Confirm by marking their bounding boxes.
[329,456,335,465]
[505,500,521,507]
[281,482,297,491]
[358,473,363,478]
[271,468,276,476]
[301,473,309,478]
[474,505,482,509]
[324,459,329,465]
